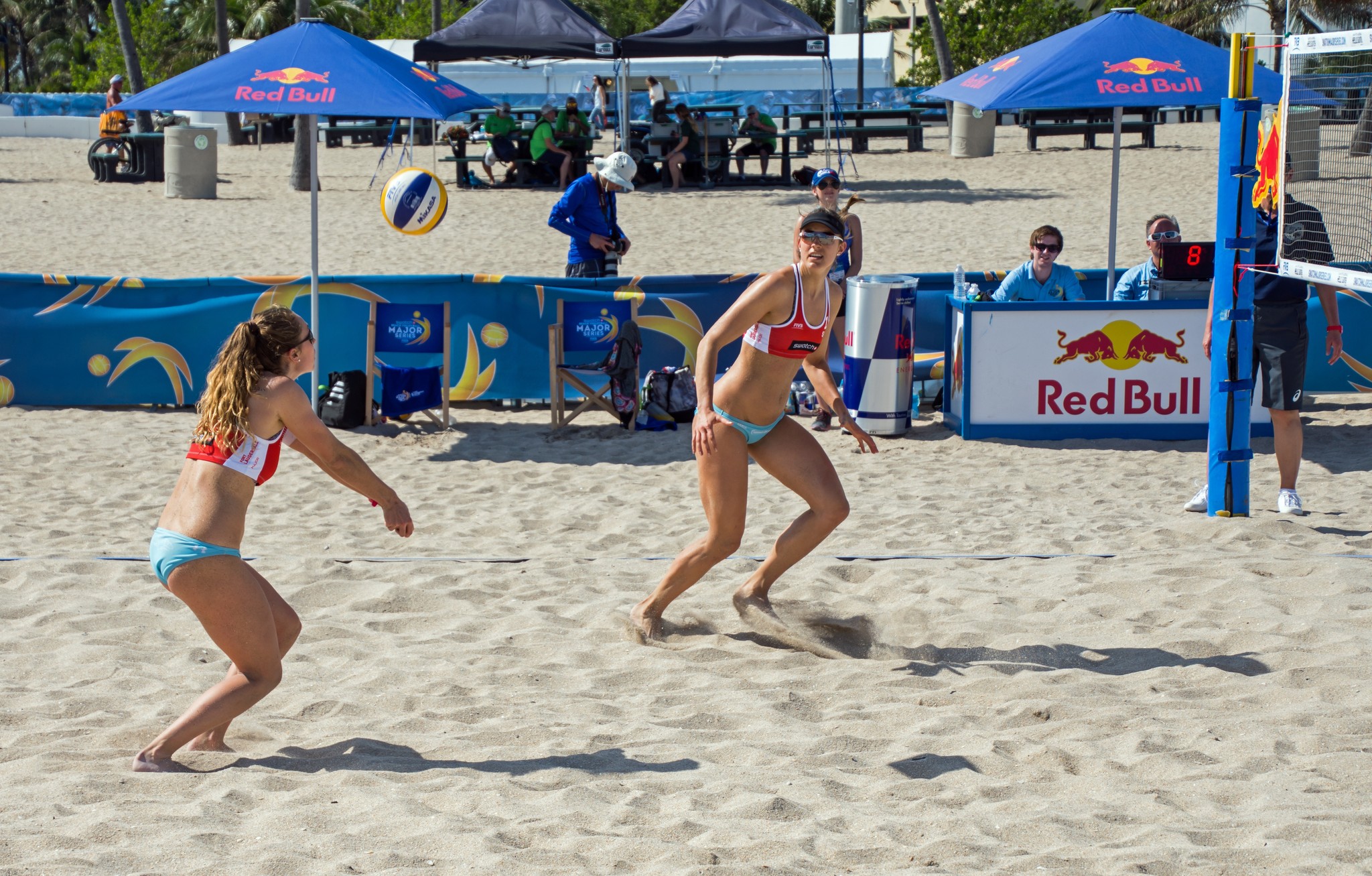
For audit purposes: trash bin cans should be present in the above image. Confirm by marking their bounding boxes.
[844,274,920,438]
[1264,106,1321,183]
[949,101,996,158]
[163,126,219,199]
[400,118,420,144]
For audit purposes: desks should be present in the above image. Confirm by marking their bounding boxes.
[449,135,602,187]
[118,132,165,182]
[1020,105,1166,151]
[908,101,1000,126]
[644,133,807,187]
[791,107,928,153]
[648,104,744,128]
[463,107,568,130]
[321,114,433,148]
[264,114,296,143]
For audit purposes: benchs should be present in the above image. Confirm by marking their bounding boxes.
[240,105,1221,189]
[91,153,119,183]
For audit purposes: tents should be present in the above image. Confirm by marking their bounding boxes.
[617,0,831,169]
[411,0,624,153]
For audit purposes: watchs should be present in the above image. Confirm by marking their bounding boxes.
[1327,325,1343,333]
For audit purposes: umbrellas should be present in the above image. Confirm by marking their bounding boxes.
[103,18,504,420]
[919,7,1346,301]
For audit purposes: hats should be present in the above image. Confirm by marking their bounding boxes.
[799,214,844,241]
[110,74,126,84]
[811,168,841,186]
[594,151,637,191]
[500,102,511,117]
[747,105,757,115]
[566,96,576,102]
[541,104,558,114]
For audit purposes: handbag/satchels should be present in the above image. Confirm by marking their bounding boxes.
[631,385,678,431]
[492,136,519,166]
[100,112,126,139]
[663,87,672,104]
[642,365,698,422]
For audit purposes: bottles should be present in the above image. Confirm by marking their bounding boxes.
[636,410,649,425]
[791,380,814,393]
[435,409,457,426]
[641,400,675,423]
[964,282,970,297]
[467,125,488,141]
[967,283,980,301]
[732,120,739,136]
[953,264,966,299]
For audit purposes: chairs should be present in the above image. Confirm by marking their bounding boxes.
[365,300,451,429]
[548,299,640,435]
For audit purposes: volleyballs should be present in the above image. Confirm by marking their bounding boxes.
[379,167,448,235]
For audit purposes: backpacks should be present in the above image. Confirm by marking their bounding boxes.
[792,165,819,186]
[317,369,378,431]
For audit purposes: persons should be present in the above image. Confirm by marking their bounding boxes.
[637,210,877,650]
[735,104,778,182]
[135,305,416,772]
[1113,213,1182,301]
[793,168,862,433]
[589,75,610,132]
[100,74,132,172]
[645,75,666,125]
[530,104,571,190]
[547,151,640,278]
[482,102,518,187]
[1185,151,1341,519]
[987,224,1087,301]
[555,96,590,172]
[665,104,699,191]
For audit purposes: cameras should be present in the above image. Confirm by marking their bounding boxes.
[603,238,625,278]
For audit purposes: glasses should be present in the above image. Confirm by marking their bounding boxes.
[818,181,840,190]
[1033,242,1060,253]
[567,102,577,107]
[1147,230,1180,241]
[279,328,314,356]
[799,230,844,246]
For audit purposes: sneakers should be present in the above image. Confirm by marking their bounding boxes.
[1183,478,1209,513]
[1277,491,1304,516]
[811,407,831,431]
